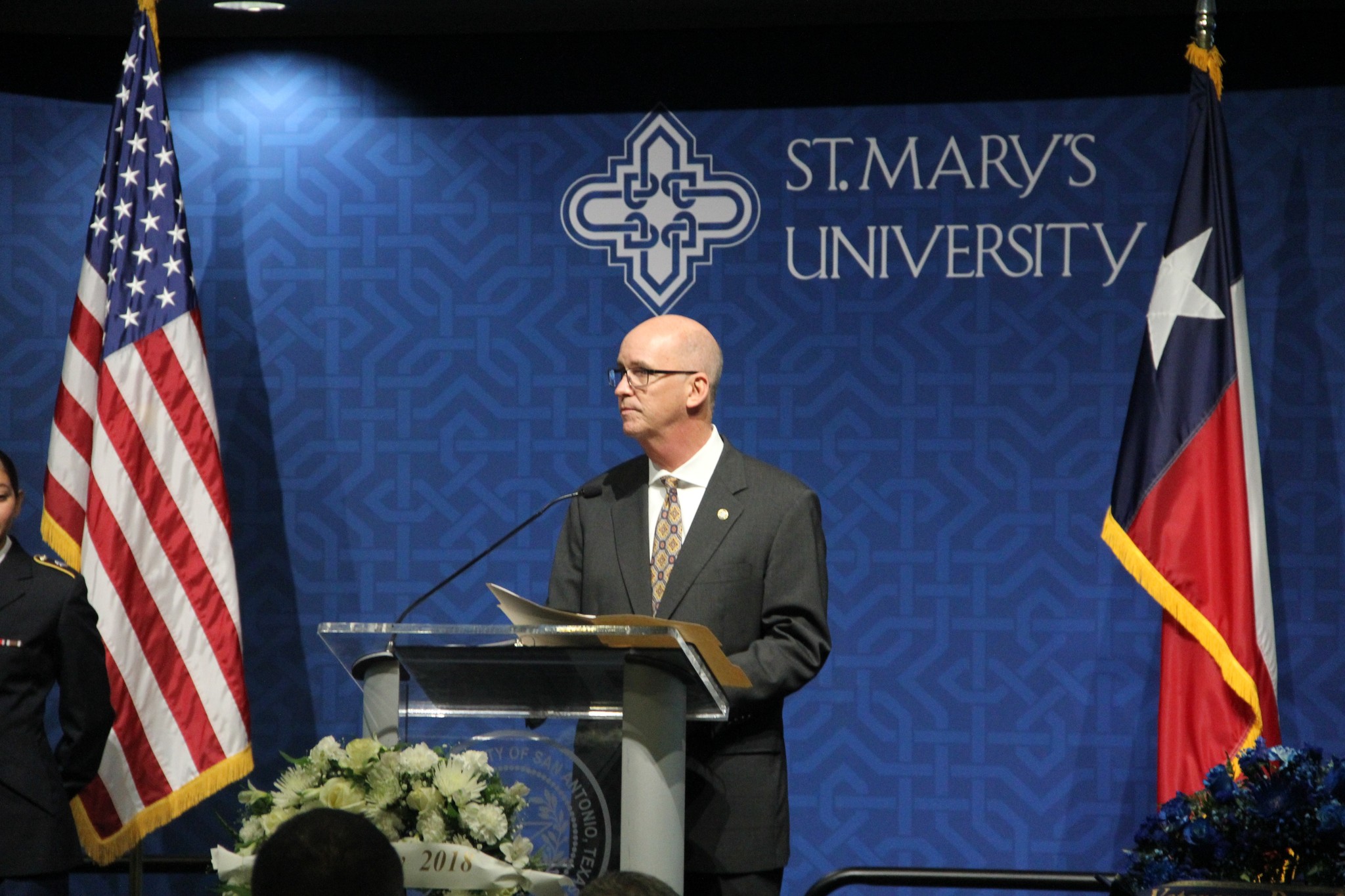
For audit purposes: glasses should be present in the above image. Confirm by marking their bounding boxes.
[606,366,698,384]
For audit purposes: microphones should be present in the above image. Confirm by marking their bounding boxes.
[395,484,602,624]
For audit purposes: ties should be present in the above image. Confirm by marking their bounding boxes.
[649,475,686,620]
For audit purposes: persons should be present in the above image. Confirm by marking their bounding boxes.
[0,449,120,895]
[573,869,680,896]
[250,809,409,896]
[523,314,831,896]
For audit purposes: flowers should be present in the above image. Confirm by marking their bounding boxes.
[208,732,569,896]
[1101,734,1345,896]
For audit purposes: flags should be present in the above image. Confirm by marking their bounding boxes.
[1100,32,1289,833]
[38,0,261,867]
